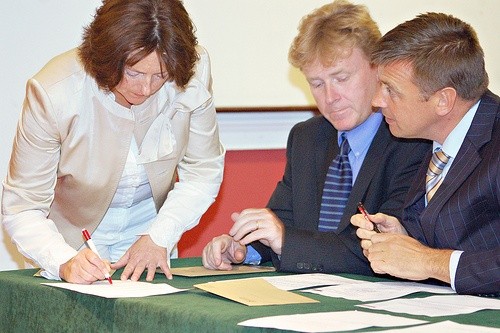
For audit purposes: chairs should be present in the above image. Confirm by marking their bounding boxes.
[178,149,287,259]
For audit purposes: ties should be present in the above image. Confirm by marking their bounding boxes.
[425,148,451,203]
[318,132,353,232]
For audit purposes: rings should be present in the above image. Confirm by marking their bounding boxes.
[256,221,259,229]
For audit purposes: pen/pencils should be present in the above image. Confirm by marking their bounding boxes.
[82,227,114,284]
[356,201,381,234]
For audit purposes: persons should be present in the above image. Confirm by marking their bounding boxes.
[350,11,500,299]
[201,0,422,273]
[0,0,226,284]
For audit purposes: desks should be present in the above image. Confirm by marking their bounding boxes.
[0,257,500,333]
